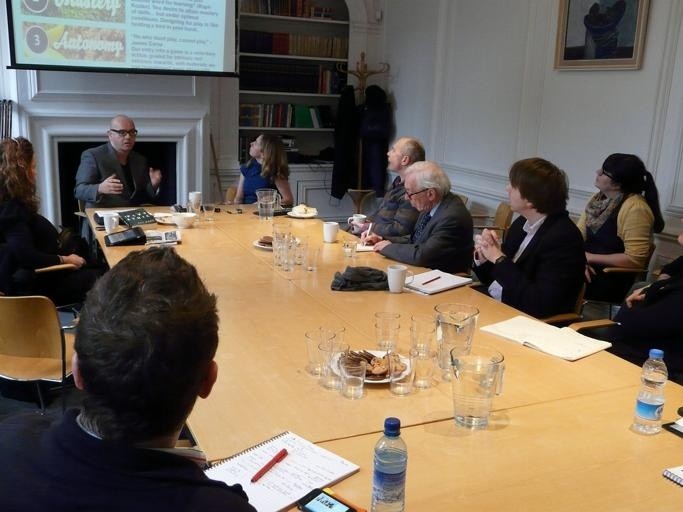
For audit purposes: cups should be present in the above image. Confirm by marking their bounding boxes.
[322,214,368,258]
[303,312,439,399]
[202,204,215,221]
[386,264,415,295]
[271,224,317,273]
[103,212,120,235]
[186,190,202,213]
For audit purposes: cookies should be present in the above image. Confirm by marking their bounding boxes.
[343,350,407,381]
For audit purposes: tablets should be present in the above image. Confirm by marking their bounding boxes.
[106,227,138,244]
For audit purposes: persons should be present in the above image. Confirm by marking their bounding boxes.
[577,152,666,304]
[348,135,426,239]
[577,227,683,388]
[361,160,474,274]
[0,246,258,512]
[220,130,295,205]
[466,157,586,318]
[0,136,110,309]
[71,113,163,264]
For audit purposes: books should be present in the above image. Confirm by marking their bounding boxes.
[480,314,613,362]
[238,0,348,163]
[202,430,362,512]
[405,267,475,297]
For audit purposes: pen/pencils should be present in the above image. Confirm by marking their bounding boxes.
[251,448,288,482]
[422,276,440,285]
[364,222,373,247]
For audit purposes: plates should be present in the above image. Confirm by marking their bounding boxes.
[286,210,318,218]
[252,238,299,250]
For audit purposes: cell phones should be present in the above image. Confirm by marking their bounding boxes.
[296,488,357,512]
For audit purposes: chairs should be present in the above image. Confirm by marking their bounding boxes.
[0,197,89,414]
[346,189,469,232]
[224,186,238,202]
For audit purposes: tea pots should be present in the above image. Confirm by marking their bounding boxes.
[253,188,281,224]
[433,304,504,429]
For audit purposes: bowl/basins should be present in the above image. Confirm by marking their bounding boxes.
[153,212,197,229]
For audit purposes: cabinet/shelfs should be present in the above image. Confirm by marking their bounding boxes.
[237,0,350,167]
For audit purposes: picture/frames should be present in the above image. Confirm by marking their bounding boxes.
[552,0,652,71]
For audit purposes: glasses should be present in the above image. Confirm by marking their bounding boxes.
[406,188,429,200]
[601,170,614,180]
[110,128,138,136]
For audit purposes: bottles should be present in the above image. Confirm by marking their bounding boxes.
[372,417,407,510]
[225,200,243,215]
[631,349,668,437]
